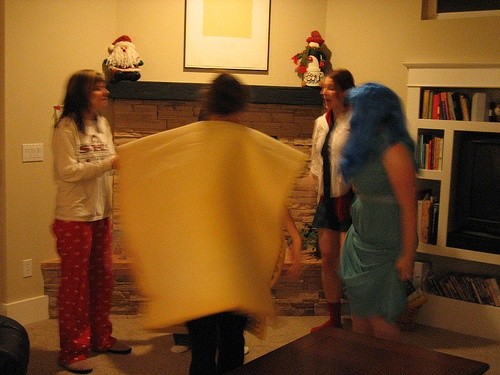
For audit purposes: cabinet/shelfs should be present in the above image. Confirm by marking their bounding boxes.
[405,63,500,340]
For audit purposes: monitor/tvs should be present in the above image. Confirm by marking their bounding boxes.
[455,140,500,240]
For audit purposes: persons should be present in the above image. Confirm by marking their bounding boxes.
[115,71,304,375]
[311,68,357,334]
[333,82,420,341]
[48,69,133,373]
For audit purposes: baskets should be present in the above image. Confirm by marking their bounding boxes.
[396,303,420,330]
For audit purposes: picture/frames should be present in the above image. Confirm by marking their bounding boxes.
[184,0,271,71]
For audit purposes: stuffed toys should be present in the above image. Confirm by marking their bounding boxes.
[103,35,144,81]
[418,191,439,245]
[291,30,327,72]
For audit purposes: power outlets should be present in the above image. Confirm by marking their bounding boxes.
[23,259,33,278]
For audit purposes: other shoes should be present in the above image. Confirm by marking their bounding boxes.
[104,341,132,354]
[170,346,188,353]
[244,345,250,355]
[59,360,93,374]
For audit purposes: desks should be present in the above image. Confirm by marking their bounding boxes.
[229,326,490,375]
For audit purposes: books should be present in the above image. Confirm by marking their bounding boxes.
[418,133,444,172]
[421,89,470,121]
[426,269,500,309]
